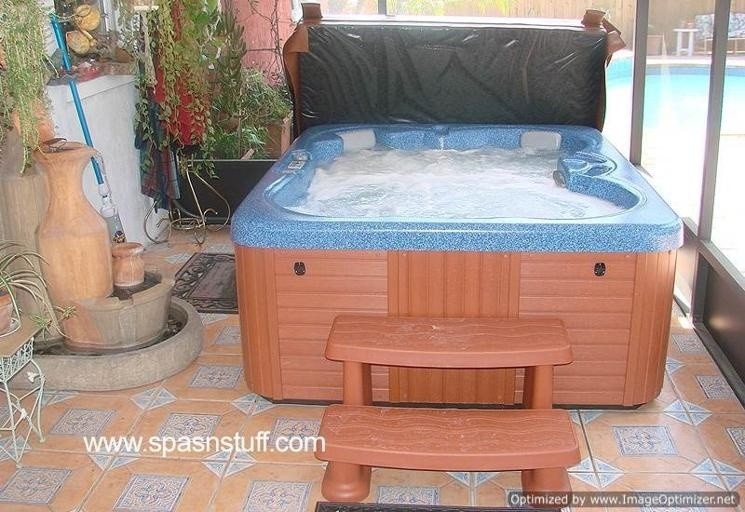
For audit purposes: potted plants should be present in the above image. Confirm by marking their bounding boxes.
[0,236,78,333]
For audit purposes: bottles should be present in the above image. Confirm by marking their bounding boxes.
[97,183,127,246]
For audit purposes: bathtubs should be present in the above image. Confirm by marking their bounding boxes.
[230,123,685,254]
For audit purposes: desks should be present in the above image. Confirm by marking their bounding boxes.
[143,144,230,244]
[1,316,45,470]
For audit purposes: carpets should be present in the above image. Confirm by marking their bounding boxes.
[170,251,239,314]
